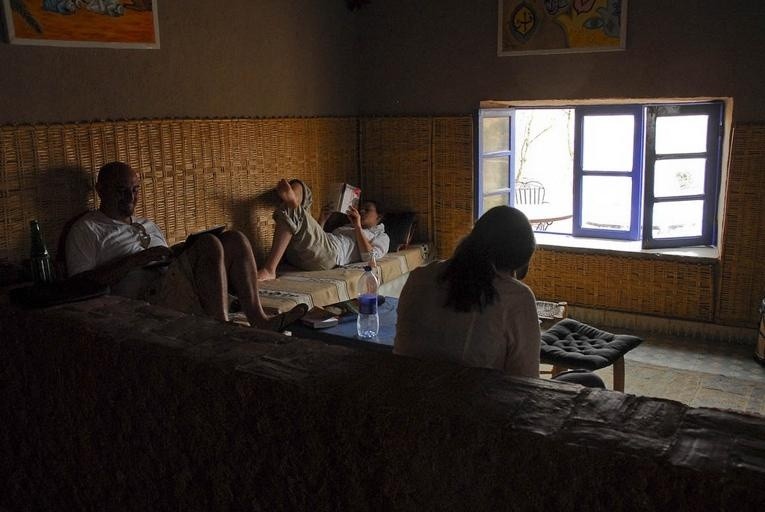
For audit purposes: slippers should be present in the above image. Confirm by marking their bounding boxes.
[276,302,308,333]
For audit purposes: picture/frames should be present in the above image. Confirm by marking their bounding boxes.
[4,0,161,49]
[496,0,627,56]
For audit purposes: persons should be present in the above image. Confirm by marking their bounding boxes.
[64,162,308,333]
[393,206,606,390]
[257,179,391,282]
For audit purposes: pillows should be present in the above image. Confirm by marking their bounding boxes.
[541,317,643,371]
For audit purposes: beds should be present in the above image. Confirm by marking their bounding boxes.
[235,242,438,332]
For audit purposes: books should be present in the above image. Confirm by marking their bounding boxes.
[327,182,361,215]
[296,306,338,329]
[322,298,361,315]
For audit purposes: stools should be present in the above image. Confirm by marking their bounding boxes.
[536,301,566,380]
[542,317,643,393]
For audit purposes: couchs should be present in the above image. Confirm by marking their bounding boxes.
[3,284,765,512]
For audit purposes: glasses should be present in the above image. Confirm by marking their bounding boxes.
[130,223,150,249]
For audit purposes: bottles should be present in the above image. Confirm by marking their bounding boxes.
[30,219,52,282]
[356,265,380,337]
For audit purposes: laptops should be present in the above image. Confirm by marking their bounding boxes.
[145,225,226,270]
